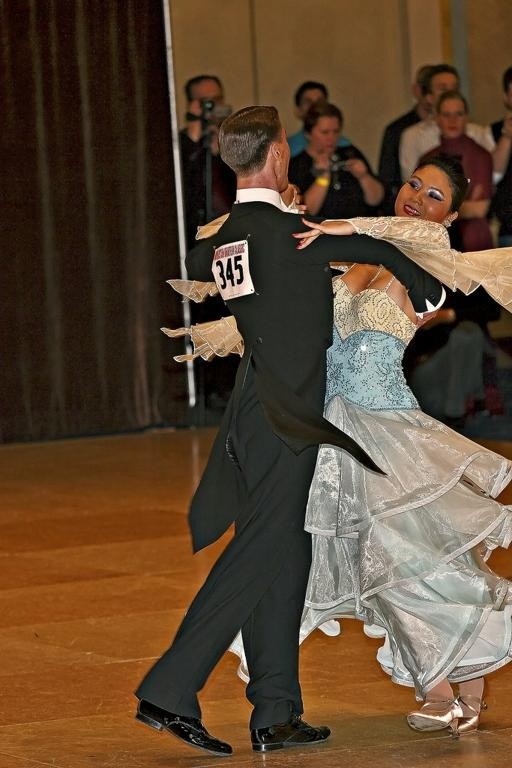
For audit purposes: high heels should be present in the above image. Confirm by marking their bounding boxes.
[458,676,488,735]
[405,698,464,740]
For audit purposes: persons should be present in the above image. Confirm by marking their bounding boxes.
[286,78,384,220]
[130,104,449,756]
[379,66,511,440]
[276,152,511,739]
[178,76,244,401]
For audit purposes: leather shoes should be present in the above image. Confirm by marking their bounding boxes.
[251,710,331,752]
[134,698,233,756]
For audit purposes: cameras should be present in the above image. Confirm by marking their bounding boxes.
[330,155,348,172]
[198,97,233,122]
